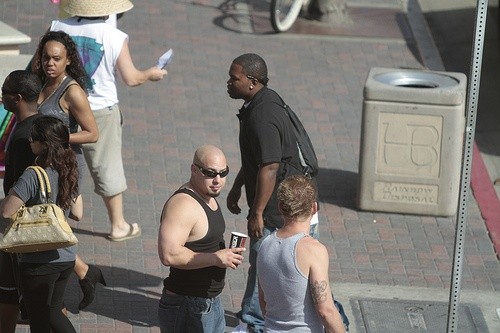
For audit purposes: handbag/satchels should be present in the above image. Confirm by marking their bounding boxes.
[0,203,79,254]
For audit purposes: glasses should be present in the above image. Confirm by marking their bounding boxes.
[194,163,230,178]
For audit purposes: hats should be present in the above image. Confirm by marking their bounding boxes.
[58,0,135,19]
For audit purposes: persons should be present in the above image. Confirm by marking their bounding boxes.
[158,145,246,333]
[256,175,346,333]
[45,0,167,242]
[226,54,350,333]
[27,31,99,171]
[0,117,83,333]
[0,69,107,333]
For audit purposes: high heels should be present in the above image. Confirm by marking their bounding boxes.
[78,264,108,311]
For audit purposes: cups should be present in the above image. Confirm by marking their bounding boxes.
[229,231,248,271]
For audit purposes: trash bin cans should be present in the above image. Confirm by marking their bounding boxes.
[358,65,468,218]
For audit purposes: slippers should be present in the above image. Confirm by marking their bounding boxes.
[108,223,142,241]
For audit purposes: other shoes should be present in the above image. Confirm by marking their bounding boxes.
[231,319,252,333]
